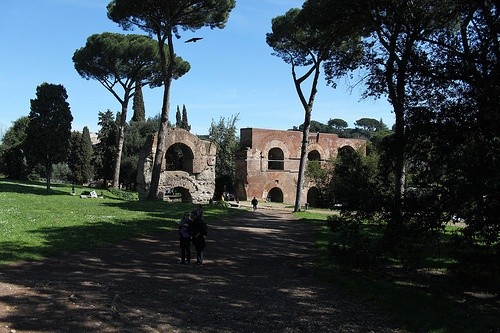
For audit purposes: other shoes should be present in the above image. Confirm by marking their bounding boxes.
[185,260,190,265]
[178,260,184,264]
[196,261,202,265]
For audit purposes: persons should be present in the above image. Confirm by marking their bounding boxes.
[179,212,193,264]
[252,197,258,208]
[190,212,208,265]
[196,204,204,221]
[222,192,230,201]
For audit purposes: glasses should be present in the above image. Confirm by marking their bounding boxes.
[185,214,189,216]
[191,215,196,216]
